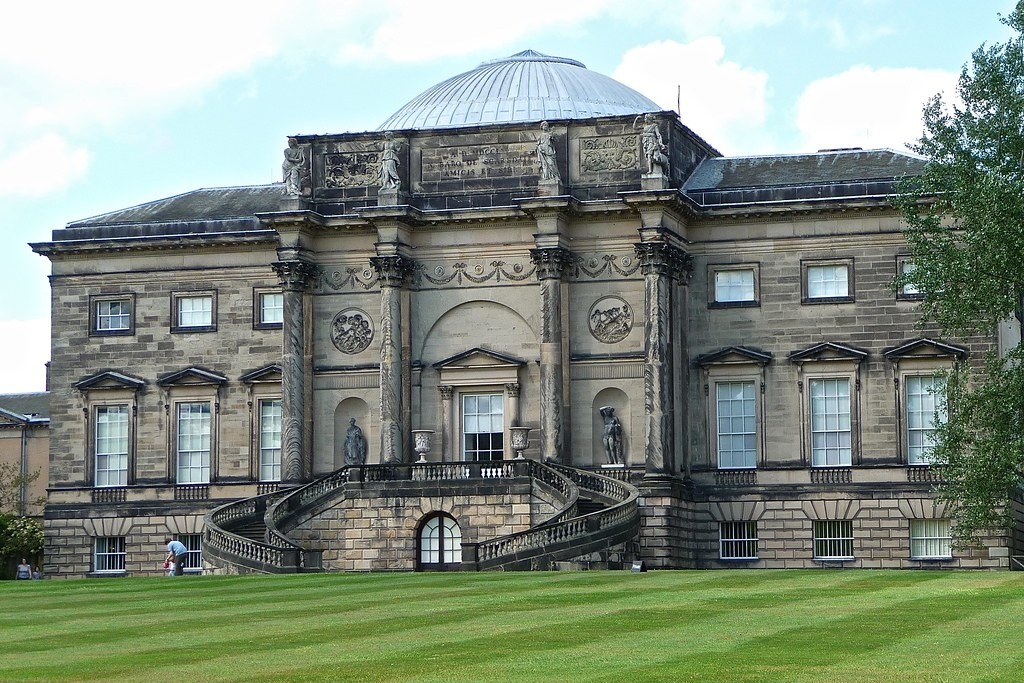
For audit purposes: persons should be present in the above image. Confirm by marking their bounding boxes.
[343,418,366,467]
[164,538,187,577]
[31,566,43,580]
[15,558,32,580]
[599,406,626,464]
